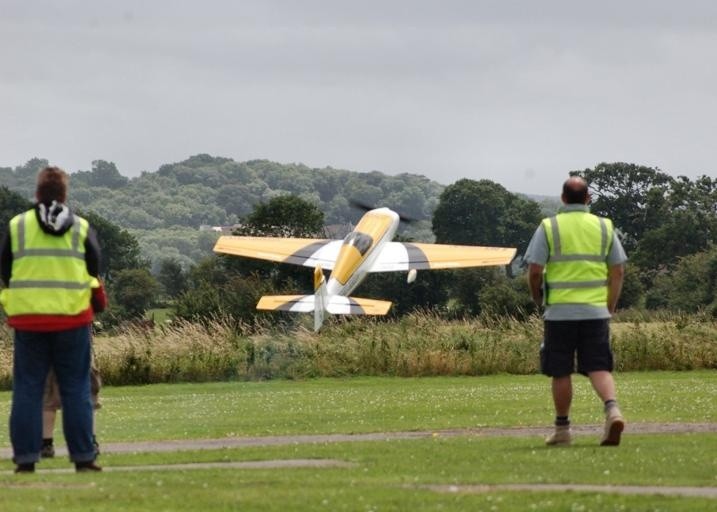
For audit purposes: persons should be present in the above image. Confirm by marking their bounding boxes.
[522,175,629,446]
[41,322,102,456]
[1,166,104,472]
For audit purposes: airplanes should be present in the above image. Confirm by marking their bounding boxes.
[211,198,521,336]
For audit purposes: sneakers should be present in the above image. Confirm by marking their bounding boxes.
[75,461,102,472]
[41,438,54,457]
[15,461,35,472]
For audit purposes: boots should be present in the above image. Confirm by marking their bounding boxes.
[600,408,624,446]
[545,426,570,444]
